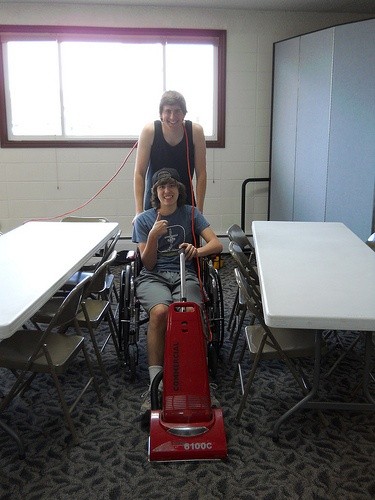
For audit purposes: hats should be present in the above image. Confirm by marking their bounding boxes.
[151,168,181,186]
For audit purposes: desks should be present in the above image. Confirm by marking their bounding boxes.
[250,220,375,442]
[0,221,120,460]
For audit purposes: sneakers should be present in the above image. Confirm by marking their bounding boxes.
[209,383,220,411]
[139,389,163,416]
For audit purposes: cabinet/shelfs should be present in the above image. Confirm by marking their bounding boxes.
[265,17,375,249]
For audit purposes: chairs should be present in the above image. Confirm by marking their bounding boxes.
[226,224,327,428]
[0,216,123,431]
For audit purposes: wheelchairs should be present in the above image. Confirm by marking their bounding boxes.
[116,249,226,379]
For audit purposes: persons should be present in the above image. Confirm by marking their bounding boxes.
[132,168,223,417]
[132,90,207,222]
